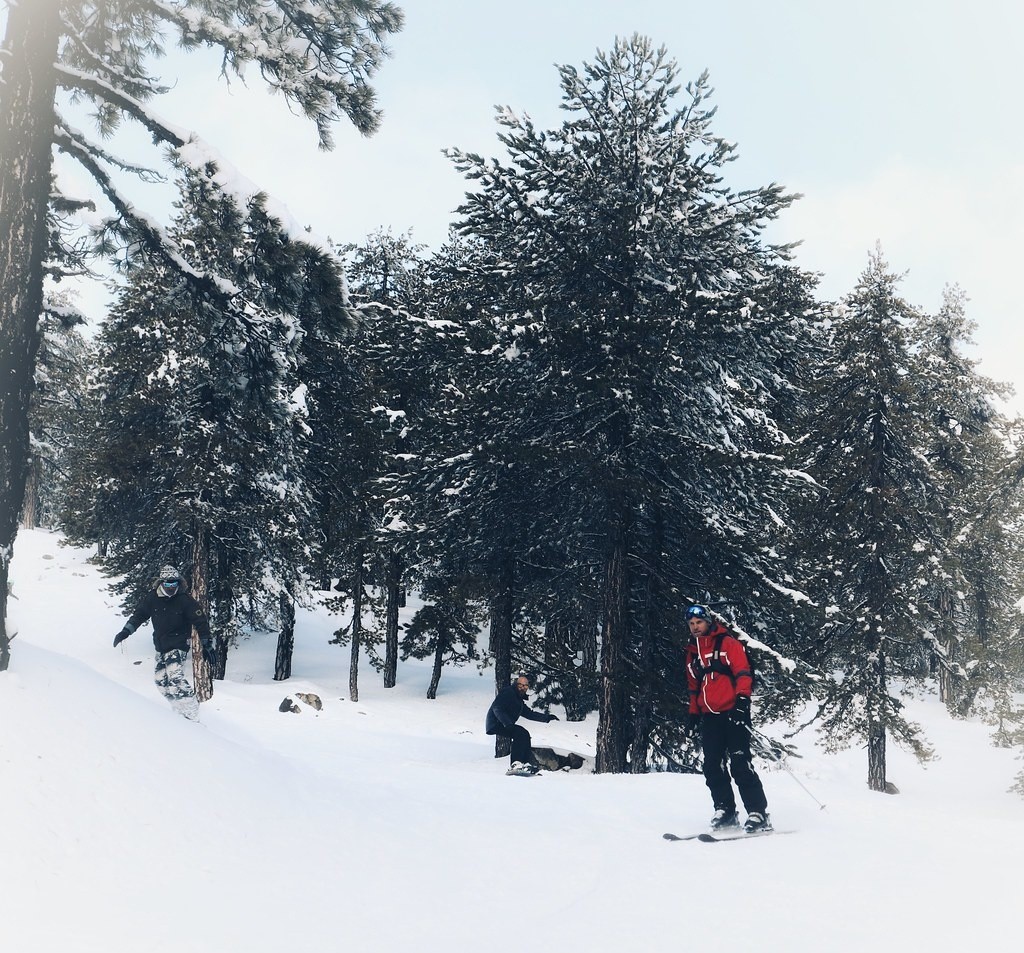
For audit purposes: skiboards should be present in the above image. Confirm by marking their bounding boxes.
[662,831,794,842]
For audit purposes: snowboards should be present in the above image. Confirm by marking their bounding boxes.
[176,712,207,727]
[505,772,543,776]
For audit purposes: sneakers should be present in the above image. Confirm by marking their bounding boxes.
[744,812,768,832]
[710,810,738,830]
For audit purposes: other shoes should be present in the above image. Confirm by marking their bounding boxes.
[509,762,539,774]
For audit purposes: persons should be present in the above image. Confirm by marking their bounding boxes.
[113,564,218,723]
[684,603,774,834]
[486,676,560,776]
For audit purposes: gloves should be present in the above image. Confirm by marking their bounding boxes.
[506,725,517,733]
[689,714,700,730]
[728,695,751,725]
[549,714,559,721]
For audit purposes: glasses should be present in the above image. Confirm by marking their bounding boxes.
[518,683,529,689]
[682,606,706,619]
